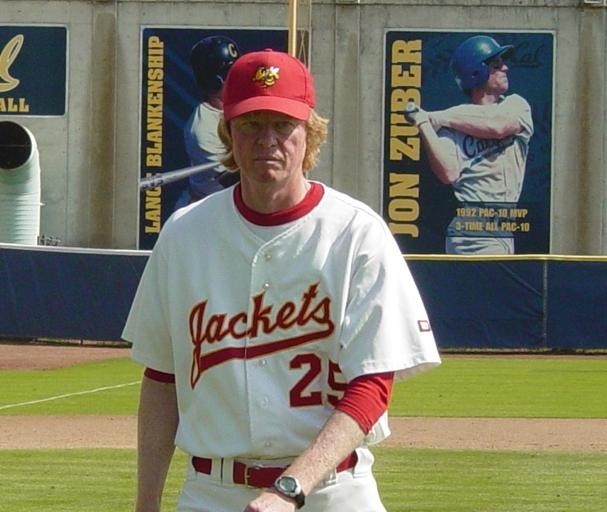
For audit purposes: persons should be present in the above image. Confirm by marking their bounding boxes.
[169,32,245,215]
[116,45,443,512]
[405,33,535,256]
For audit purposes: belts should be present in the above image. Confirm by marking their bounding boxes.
[185,446,358,491]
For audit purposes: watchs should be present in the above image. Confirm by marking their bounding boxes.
[269,475,305,510]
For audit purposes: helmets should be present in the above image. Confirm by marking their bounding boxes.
[188,35,240,94]
[448,33,519,98]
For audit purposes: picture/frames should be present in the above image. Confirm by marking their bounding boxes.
[0,21,70,119]
[136,25,313,250]
[380,28,556,254]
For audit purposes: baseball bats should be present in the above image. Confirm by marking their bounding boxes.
[139,162,221,190]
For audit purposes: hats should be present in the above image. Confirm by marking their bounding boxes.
[218,46,318,120]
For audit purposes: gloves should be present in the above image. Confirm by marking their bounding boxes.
[426,107,449,131]
[403,105,429,129]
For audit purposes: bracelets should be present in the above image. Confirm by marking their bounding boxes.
[415,119,429,128]
